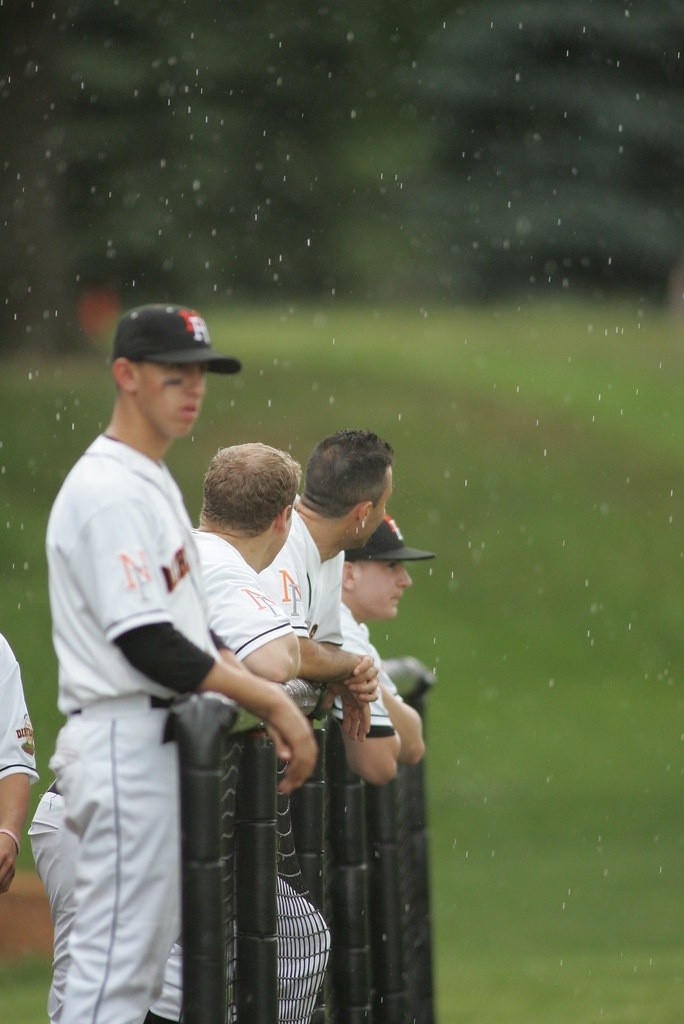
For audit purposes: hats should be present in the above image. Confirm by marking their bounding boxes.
[345,513,435,560]
[112,303,241,374]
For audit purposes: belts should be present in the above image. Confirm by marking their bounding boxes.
[47,780,64,796]
[71,695,173,715]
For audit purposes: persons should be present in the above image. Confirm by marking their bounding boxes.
[21,442,329,1010]
[0,628,40,897]
[340,515,425,768]
[42,306,317,1024]
[261,427,383,743]
